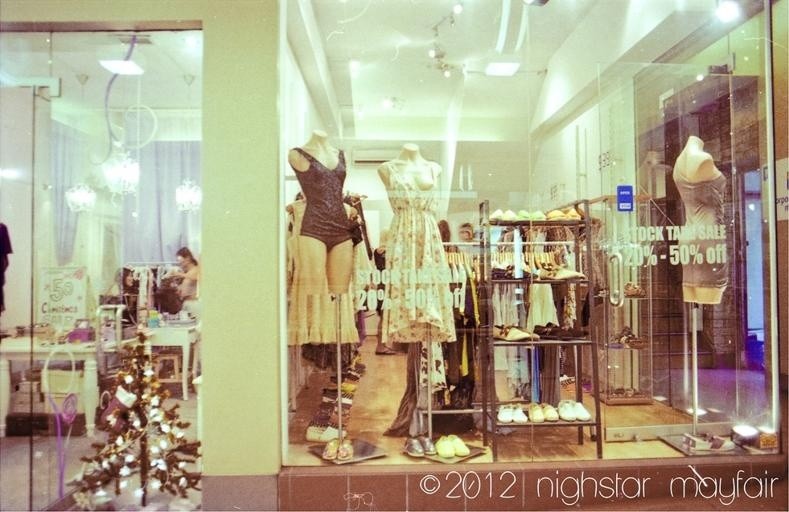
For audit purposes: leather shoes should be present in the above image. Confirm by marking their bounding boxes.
[306,363,365,461]
[599,381,635,397]
[493,322,586,342]
[683,432,734,450]
[491,265,586,280]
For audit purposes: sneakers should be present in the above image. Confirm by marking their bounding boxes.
[403,435,470,457]
[498,400,591,424]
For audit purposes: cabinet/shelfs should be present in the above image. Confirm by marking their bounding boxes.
[477,200,605,462]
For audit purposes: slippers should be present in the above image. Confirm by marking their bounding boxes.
[489,209,580,221]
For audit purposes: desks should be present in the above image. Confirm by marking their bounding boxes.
[0,322,198,440]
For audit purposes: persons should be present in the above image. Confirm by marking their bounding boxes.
[374,225,400,356]
[161,246,200,392]
[288,129,354,297]
[459,222,475,242]
[102,267,133,312]
[672,134,727,305]
[377,141,459,347]
[438,219,459,255]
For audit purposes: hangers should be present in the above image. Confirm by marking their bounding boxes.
[443,249,559,270]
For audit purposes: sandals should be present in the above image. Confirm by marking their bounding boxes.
[376,343,395,354]
